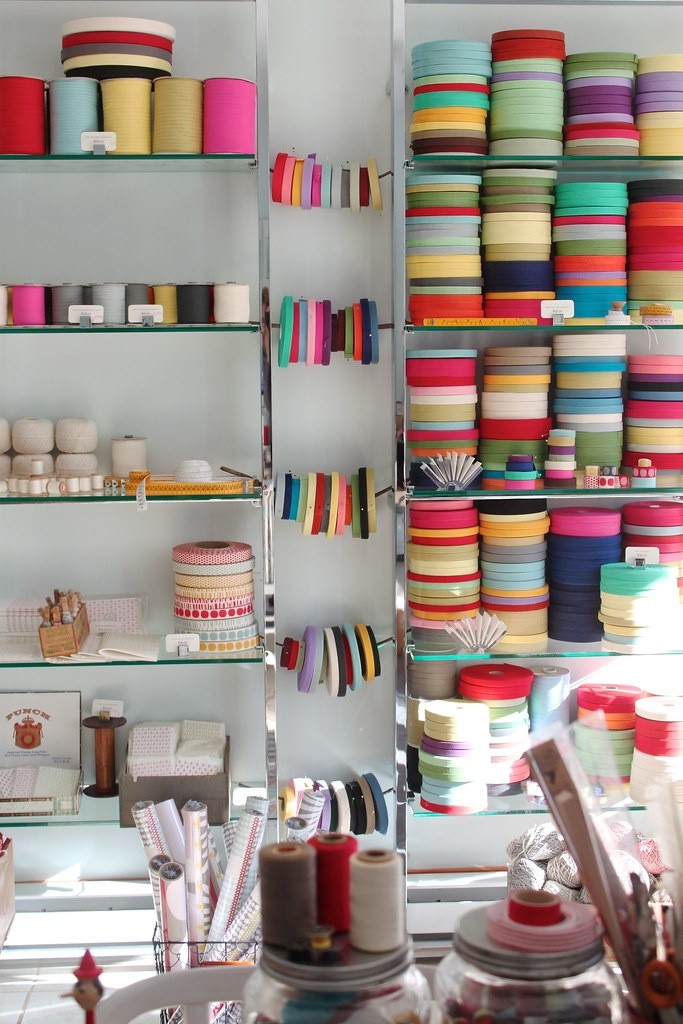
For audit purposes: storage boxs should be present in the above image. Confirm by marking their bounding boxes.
[116,735,231,828]
[0,769,85,818]
[0,838,16,951]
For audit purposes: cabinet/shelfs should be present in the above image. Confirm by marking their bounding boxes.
[0,0,279,952]
[389,0,683,904]
